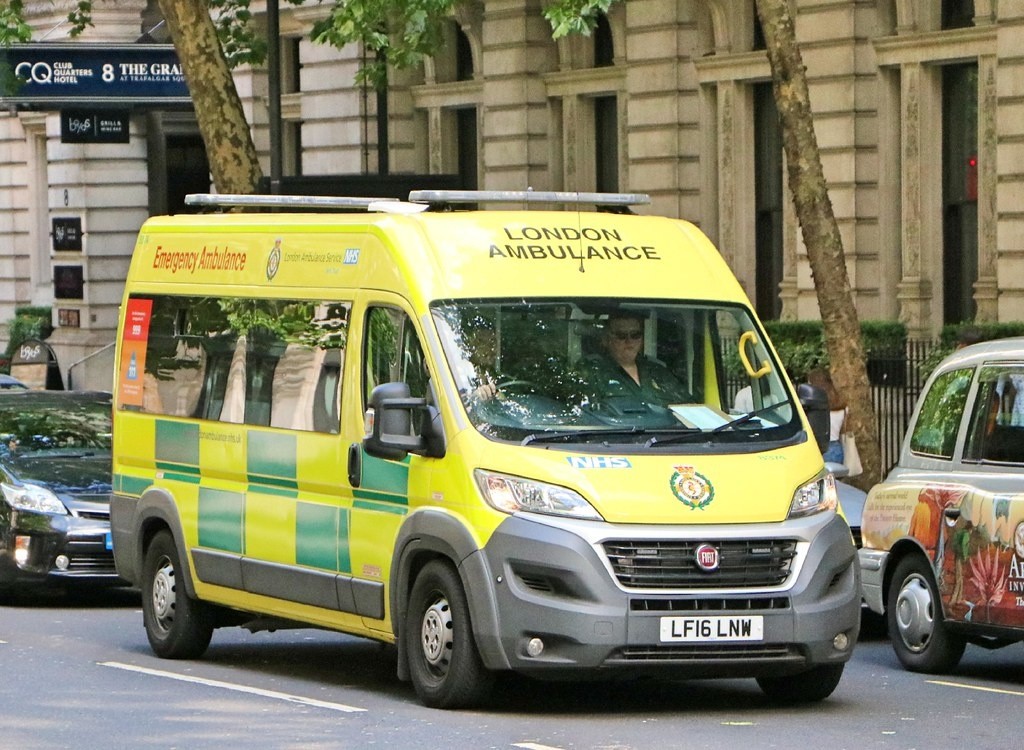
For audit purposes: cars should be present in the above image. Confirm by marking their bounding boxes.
[2,389,132,605]
[857,335,1024,675]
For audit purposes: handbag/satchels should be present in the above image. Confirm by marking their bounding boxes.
[839,407,863,478]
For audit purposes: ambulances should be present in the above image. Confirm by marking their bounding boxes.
[108,189,862,711]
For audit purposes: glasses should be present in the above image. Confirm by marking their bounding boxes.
[611,330,643,341]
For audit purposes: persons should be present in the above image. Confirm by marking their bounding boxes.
[565,311,699,414]
[421,315,525,412]
[982,389,1007,463]
